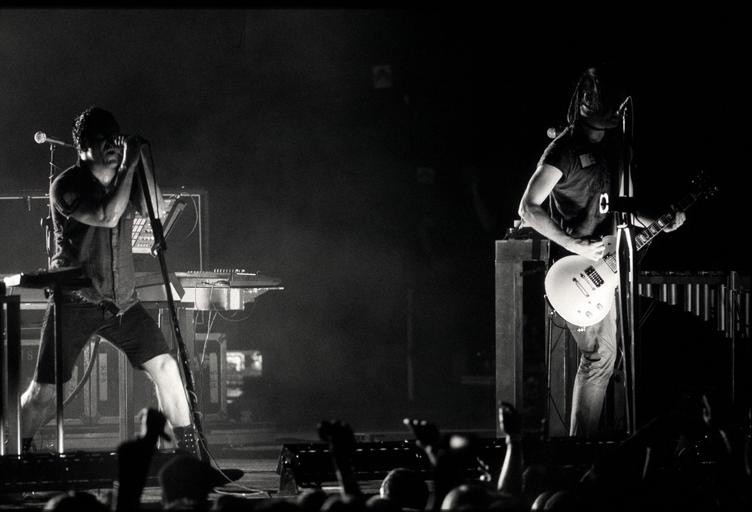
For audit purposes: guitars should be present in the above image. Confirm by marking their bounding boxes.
[544,171,719,327]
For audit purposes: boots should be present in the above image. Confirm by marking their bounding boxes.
[174,425,245,486]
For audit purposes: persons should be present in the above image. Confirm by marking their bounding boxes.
[21,104,202,466]
[1,399,752,511]
[517,56,688,439]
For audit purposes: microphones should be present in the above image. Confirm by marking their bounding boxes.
[614,96,630,119]
[34,131,73,148]
